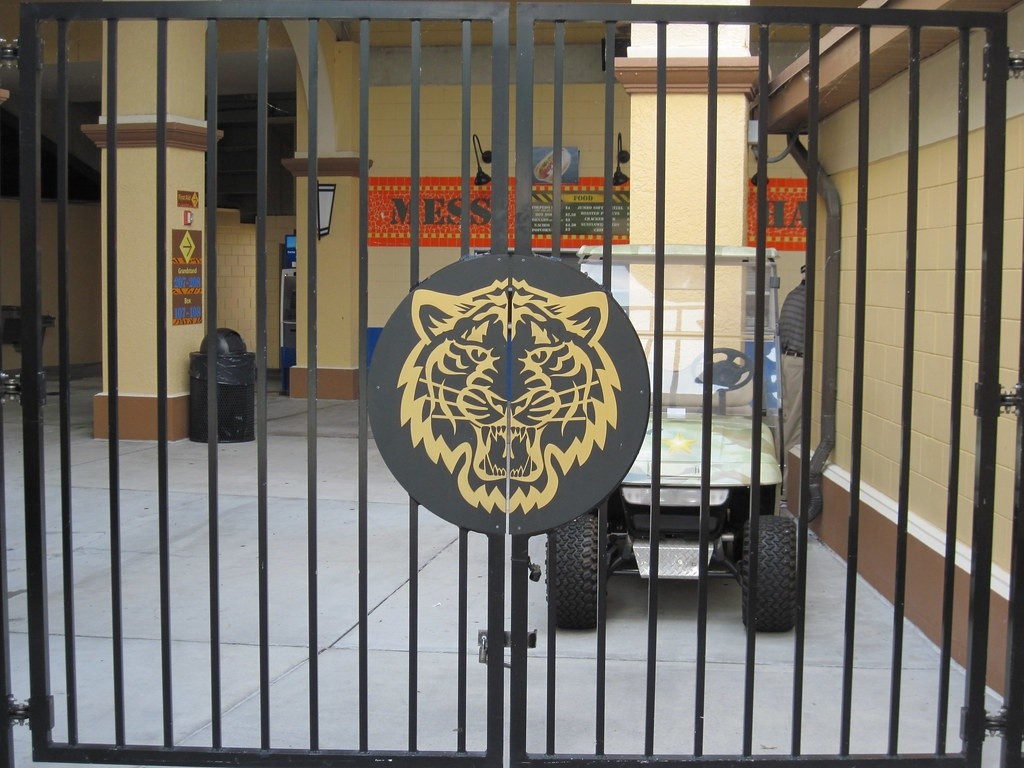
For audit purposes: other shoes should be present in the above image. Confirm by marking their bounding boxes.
[780,498,787,509]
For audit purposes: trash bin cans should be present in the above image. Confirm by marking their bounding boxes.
[185,326,257,444]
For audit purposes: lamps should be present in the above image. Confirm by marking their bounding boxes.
[613,132,629,185]
[473,134,491,185]
[317,179,336,240]
[751,173,769,187]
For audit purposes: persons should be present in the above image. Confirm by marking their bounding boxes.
[778,266,806,478]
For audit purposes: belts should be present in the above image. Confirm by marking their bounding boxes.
[781,350,805,358]
